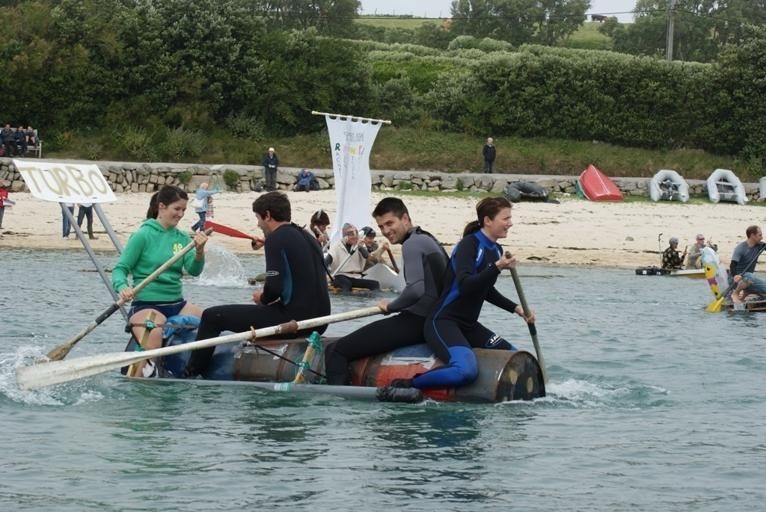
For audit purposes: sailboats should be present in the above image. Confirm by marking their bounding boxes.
[311,110,393,296]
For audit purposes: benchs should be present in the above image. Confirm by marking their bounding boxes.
[0,128,43,158]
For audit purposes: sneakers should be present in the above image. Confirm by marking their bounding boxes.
[391,379,415,388]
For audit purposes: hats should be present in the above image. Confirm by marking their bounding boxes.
[342,223,356,232]
[312,211,330,225]
[269,147,274,152]
[362,227,376,237]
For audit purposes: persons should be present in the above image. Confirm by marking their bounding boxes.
[294,169,314,192]
[688,233,717,268]
[324,197,450,386]
[184,192,330,379]
[307,210,389,296]
[482,138,496,173]
[191,183,225,231]
[62,203,74,238]
[663,237,688,268]
[729,225,766,297]
[391,197,535,388]
[111,185,208,361]
[0,124,39,157]
[0,184,8,229]
[75,203,98,239]
[262,147,278,190]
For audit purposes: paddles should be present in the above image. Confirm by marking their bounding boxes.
[16,307,385,386]
[204,221,266,246]
[45,229,214,359]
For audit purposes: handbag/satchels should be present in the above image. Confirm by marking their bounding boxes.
[190,197,204,208]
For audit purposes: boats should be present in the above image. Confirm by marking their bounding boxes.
[649,170,690,203]
[577,164,624,201]
[509,180,549,202]
[707,168,749,205]
[635,264,730,280]
